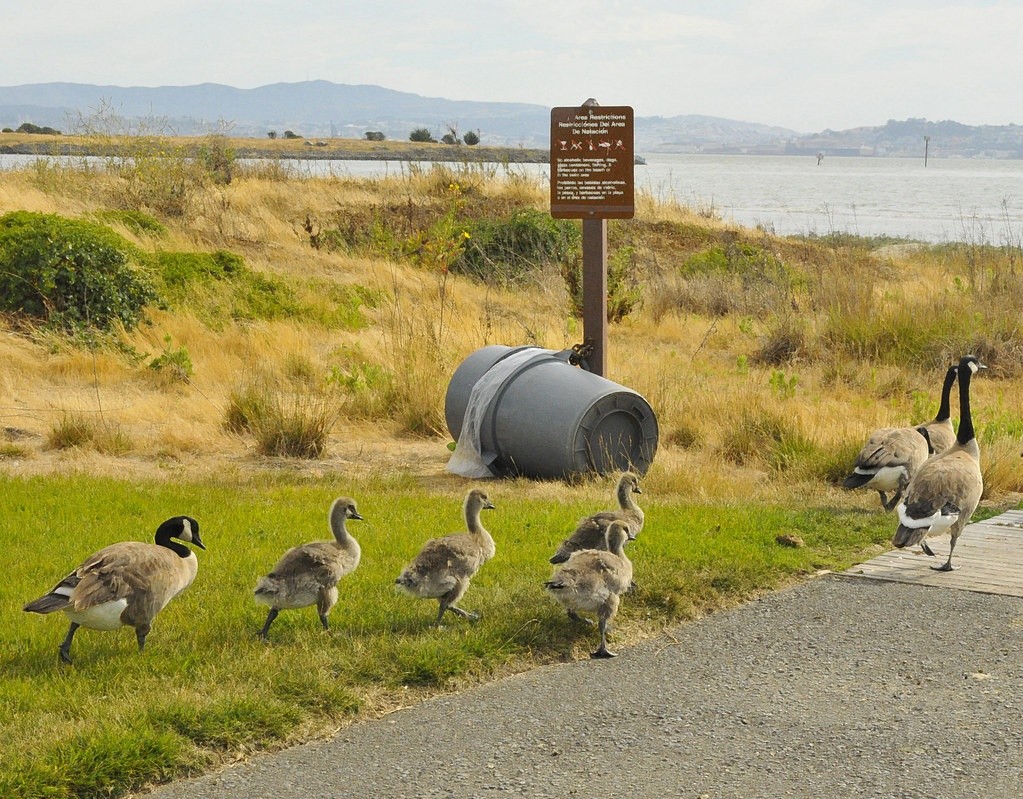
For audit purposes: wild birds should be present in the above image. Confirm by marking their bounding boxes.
[542,520,638,658]
[21,516,206,665]
[392,489,497,631]
[548,472,645,587]
[891,353,989,572]
[252,496,366,641]
[841,363,958,517]
